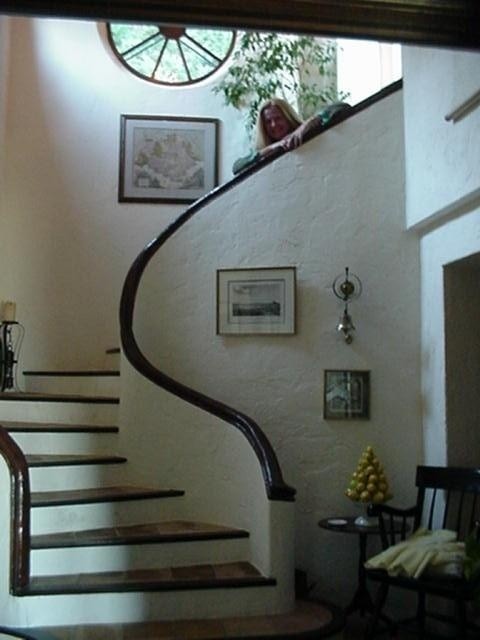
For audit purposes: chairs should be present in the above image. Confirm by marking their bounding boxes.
[361,462,480,639]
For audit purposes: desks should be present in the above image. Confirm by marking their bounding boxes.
[316,513,416,640]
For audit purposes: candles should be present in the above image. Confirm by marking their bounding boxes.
[1,298,18,322]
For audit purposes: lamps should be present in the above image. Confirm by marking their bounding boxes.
[327,266,366,345]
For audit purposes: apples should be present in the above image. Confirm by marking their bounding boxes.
[345,445,389,502]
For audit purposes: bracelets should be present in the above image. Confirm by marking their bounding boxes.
[306,119,315,129]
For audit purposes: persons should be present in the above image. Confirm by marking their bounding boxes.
[233,98,351,175]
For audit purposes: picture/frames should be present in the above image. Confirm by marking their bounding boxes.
[214,264,299,339]
[116,111,225,205]
[323,367,373,423]
